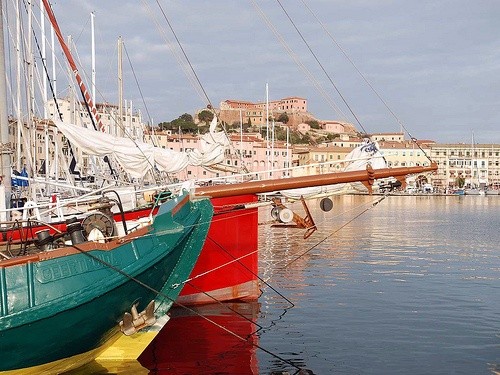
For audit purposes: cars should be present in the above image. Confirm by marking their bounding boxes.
[451,189,467,195]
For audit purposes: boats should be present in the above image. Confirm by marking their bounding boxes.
[0,1,439,375]
